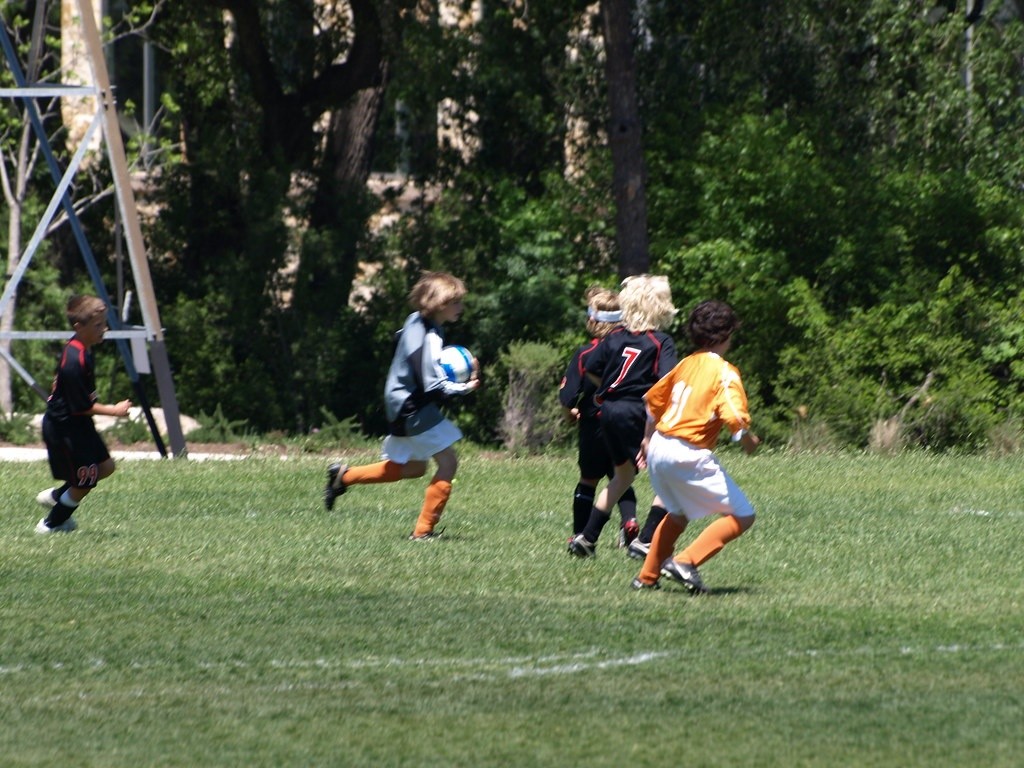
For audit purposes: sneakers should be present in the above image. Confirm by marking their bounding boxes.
[409,530,439,542]
[324,461,349,511]
[630,578,662,591]
[660,555,707,593]
[36,518,77,533]
[35,488,58,508]
[569,533,596,558]
[627,537,651,561]
[619,518,639,547]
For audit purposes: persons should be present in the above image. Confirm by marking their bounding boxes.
[35,293,132,535]
[559,289,640,548]
[566,273,678,558]
[632,300,759,591]
[323,273,480,543]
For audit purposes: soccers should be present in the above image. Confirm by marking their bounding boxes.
[439,344,473,384]
